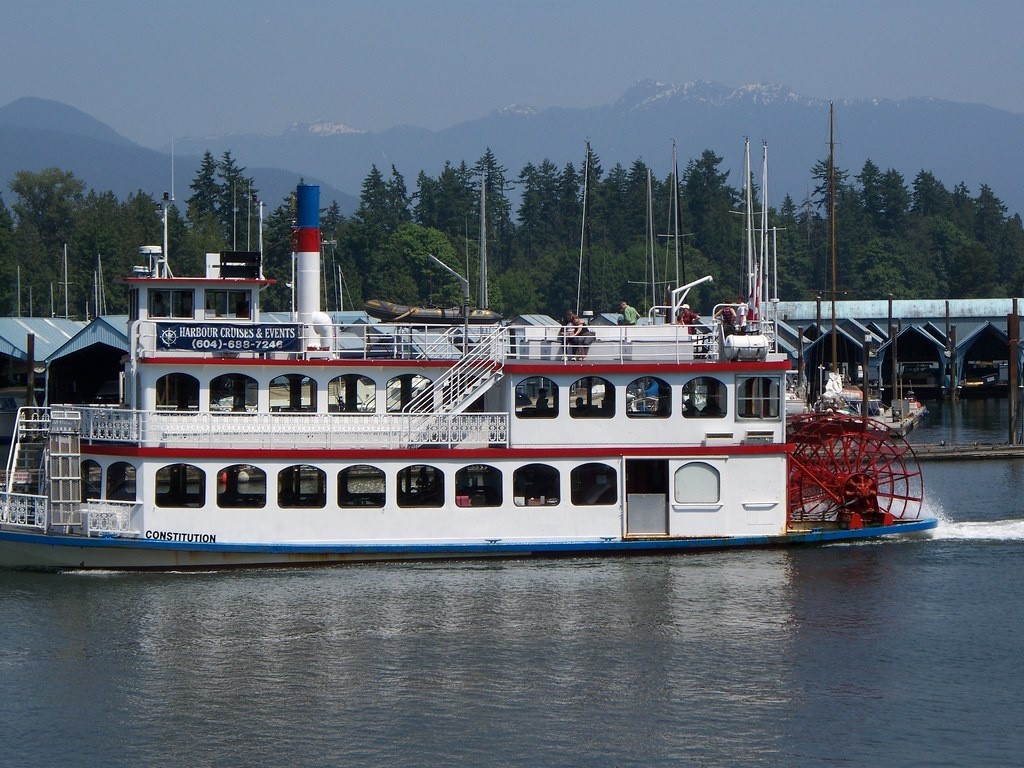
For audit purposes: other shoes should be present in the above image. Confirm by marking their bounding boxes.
[561,356,568,361]
[571,357,576,361]
[578,357,587,361]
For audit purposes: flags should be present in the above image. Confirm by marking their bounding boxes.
[747,278,760,320]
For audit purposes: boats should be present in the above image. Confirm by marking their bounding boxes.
[0,183,942,574]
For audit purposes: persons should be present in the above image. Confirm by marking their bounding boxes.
[536,388,549,408]
[558,312,576,361]
[676,304,701,334]
[570,316,595,360]
[713,294,749,340]
[574,397,586,408]
[617,300,640,325]
[684,400,700,416]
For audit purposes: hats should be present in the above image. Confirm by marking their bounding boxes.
[538,388,549,395]
[618,301,624,308]
[732,294,743,299]
[680,304,690,309]
